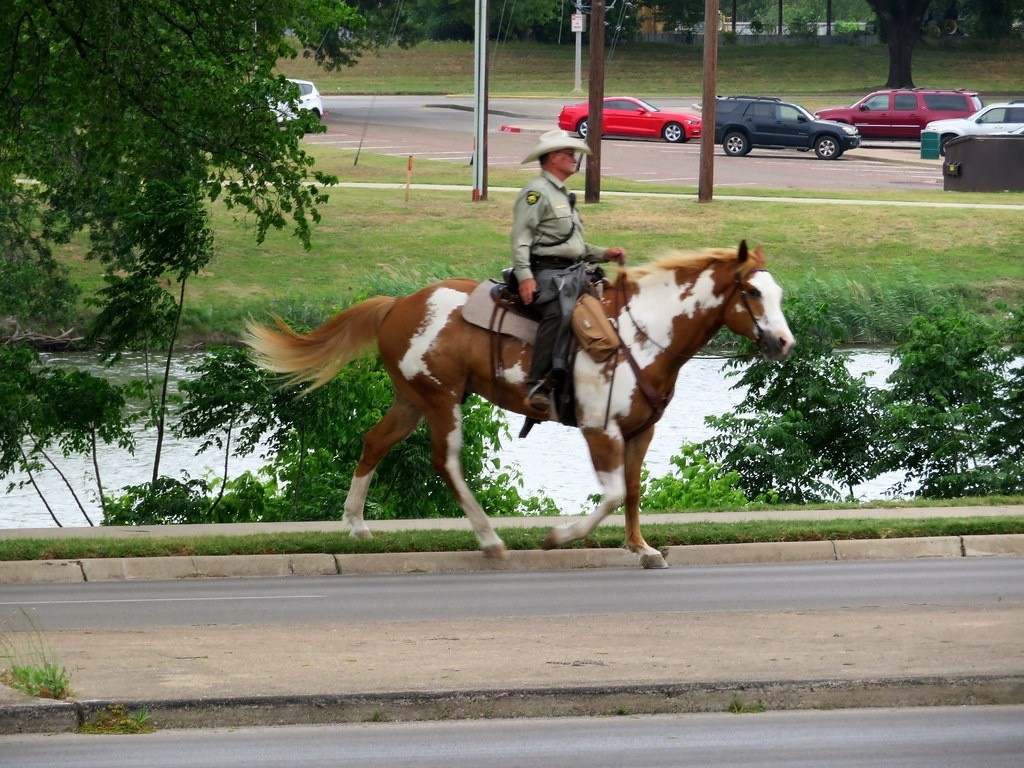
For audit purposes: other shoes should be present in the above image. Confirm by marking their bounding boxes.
[524,391,551,411]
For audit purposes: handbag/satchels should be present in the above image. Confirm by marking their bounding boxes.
[571,294,621,363]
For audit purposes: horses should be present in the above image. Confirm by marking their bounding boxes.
[241,240,795,568]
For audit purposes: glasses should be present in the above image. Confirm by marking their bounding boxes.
[556,150,575,158]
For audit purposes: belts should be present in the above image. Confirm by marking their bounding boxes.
[550,257,574,265]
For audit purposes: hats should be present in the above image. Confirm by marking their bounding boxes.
[520,130,593,165]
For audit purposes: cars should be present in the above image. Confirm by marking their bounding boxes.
[558,97,702,143]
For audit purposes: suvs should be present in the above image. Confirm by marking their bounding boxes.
[814,87,984,140]
[715,95,861,160]
[925,99,1024,157]
[263,79,324,124]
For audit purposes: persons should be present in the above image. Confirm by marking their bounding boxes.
[511,130,628,411]
